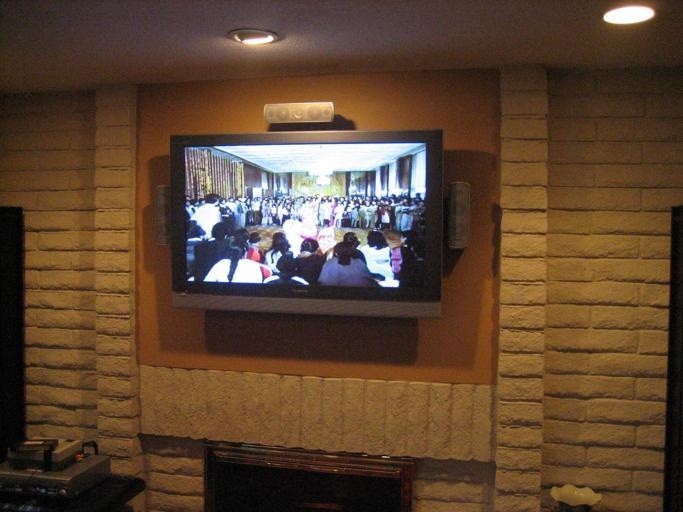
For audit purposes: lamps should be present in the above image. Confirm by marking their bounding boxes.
[222,26,280,47]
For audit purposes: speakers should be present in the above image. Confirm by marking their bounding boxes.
[150,184,171,245]
[263,100,334,124]
[447,180,472,250]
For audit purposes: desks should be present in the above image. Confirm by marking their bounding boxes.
[0,469,148,512]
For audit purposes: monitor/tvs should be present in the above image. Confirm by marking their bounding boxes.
[169,129,444,319]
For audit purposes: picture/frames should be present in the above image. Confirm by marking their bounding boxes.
[202,438,417,511]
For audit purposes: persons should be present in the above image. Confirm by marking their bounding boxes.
[184,192,425,286]
